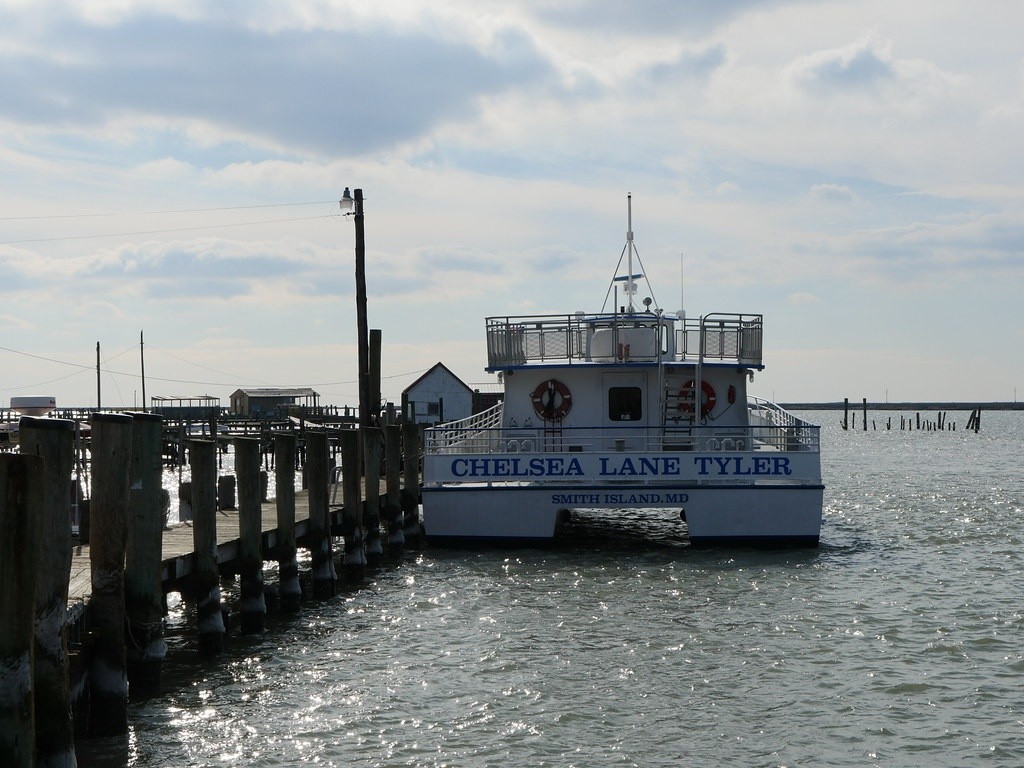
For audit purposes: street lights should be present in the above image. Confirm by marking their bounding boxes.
[340,188,373,472]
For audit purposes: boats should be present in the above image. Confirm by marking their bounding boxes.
[421,192,826,550]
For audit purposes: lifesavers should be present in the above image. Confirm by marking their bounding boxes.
[677,379,716,418]
[532,380,571,419]
